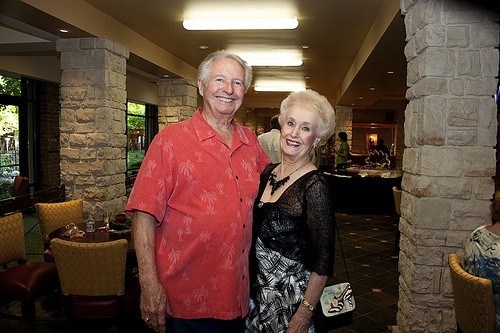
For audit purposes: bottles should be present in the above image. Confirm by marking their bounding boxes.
[86,214,95,233]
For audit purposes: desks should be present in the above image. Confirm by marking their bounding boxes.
[48,220,135,257]
[324,167,401,216]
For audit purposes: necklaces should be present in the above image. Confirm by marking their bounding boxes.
[268,158,310,195]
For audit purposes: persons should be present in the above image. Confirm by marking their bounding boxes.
[462,190,500,333]
[333,131,349,173]
[130,50,272,333]
[369,138,394,167]
[242,89,336,333]
[257,114,283,166]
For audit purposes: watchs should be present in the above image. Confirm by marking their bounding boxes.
[301,299,317,311]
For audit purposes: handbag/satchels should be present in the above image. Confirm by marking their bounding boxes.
[314,282,357,331]
[347,152,351,160]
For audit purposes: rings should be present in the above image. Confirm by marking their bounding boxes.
[144,315,151,321]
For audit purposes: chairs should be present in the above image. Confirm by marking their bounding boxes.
[0,212,61,333]
[50,238,140,333]
[448,253,494,333]
[392,187,402,247]
[34,198,85,261]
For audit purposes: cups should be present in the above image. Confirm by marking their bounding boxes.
[69,223,78,236]
[103,212,110,229]
